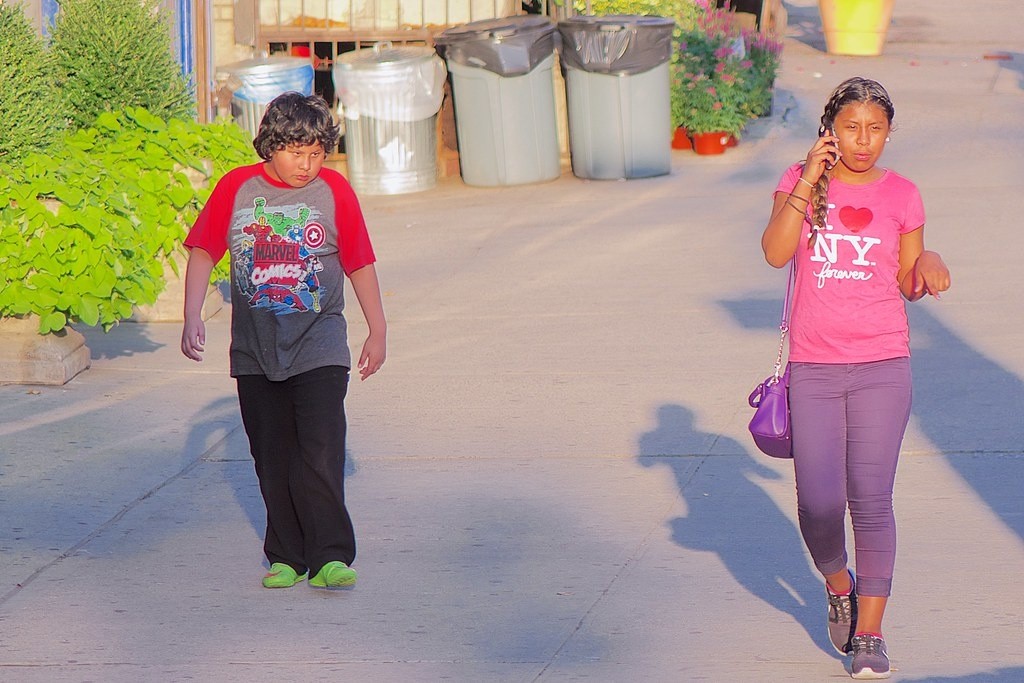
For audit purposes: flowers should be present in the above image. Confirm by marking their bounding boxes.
[667,0,782,143]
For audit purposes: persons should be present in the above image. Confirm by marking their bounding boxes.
[761,76,951,678]
[182,91,387,587]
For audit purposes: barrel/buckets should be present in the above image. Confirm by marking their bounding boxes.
[818,0,898,58]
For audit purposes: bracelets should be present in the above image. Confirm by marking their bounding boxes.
[790,193,809,204]
[785,200,806,215]
[799,178,814,187]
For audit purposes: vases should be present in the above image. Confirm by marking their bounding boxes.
[670,125,740,156]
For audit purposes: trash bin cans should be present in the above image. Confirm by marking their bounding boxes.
[434,14,562,187]
[333,41,438,197]
[214,50,315,141]
[559,16,675,180]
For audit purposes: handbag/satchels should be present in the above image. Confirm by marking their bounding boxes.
[748,369,794,462]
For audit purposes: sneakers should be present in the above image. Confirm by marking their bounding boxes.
[849,625,892,681]
[307,559,358,589]
[825,566,858,657]
[262,560,308,587]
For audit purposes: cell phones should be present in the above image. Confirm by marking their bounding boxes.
[818,122,840,170]
